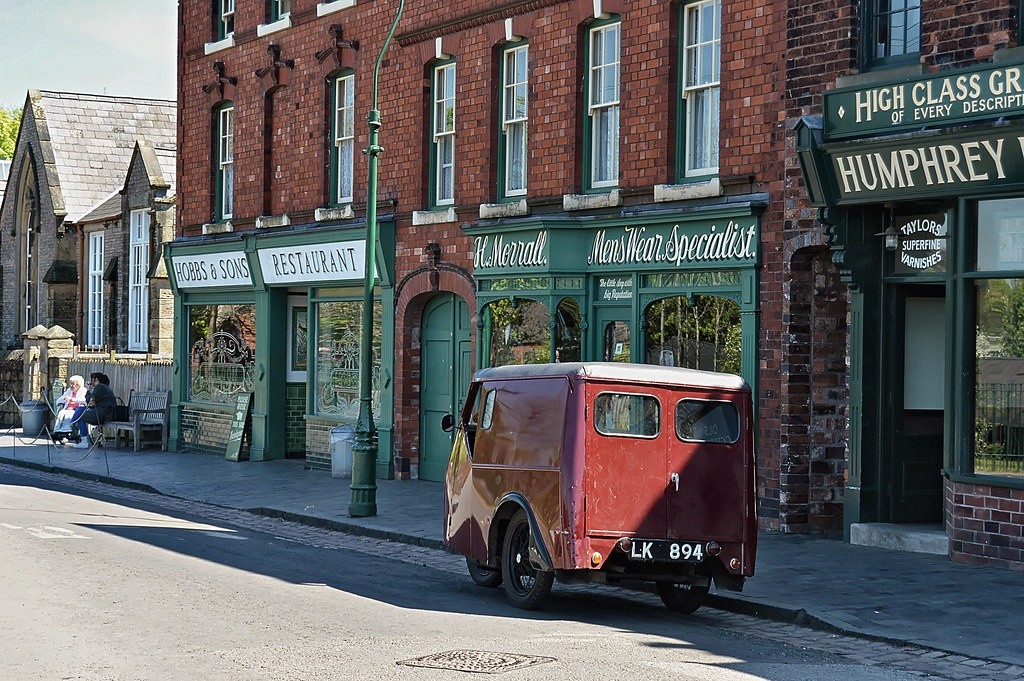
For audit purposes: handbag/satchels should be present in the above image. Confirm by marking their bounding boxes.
[112,395,129,422]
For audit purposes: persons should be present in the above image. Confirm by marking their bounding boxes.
[70,371,120,449]
[49,375,95,445]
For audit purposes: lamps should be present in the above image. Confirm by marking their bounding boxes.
[874,201,908,253]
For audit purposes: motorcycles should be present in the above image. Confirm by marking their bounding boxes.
[440,359,760,616]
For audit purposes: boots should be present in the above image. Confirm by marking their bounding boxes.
[73,436,91,449]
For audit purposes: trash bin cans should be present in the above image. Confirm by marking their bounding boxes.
[19,399,49,436]
[328,422,360,479]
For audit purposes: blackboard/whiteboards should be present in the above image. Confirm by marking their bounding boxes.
[224,391,255,462]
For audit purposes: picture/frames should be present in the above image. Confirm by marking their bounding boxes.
[290,305,308,372]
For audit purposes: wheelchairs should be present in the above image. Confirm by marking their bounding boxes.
[51,403,99,445]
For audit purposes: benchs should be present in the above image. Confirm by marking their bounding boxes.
[96,389,173,452]
